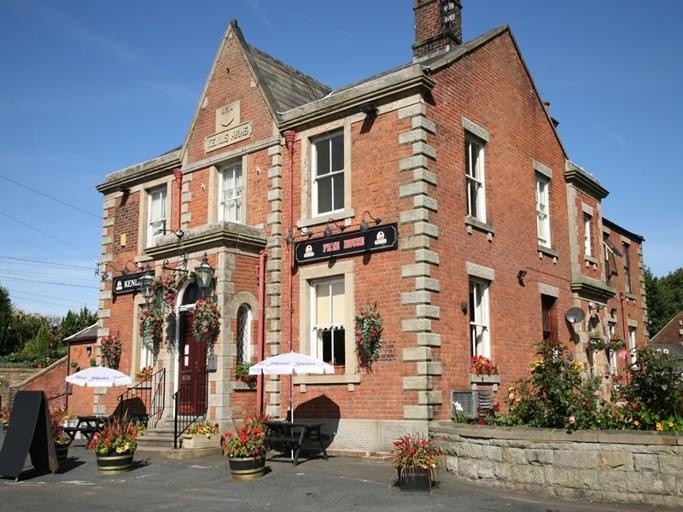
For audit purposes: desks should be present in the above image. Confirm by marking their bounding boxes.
[71,416,104,440]
[264,422,329,464]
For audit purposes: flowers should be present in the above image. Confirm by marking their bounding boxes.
[191,296,221,344]
[385,432,444,485]
[86,413,140,453]
[354,304,384,367]
[472,356,496,375]
[217,415,273,459]
[137,268,177,338]
[51,415,68,443]
[99,334,124,368]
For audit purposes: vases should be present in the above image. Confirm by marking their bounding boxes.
[397,461,432,490]
[227,453,267,480]
[96,449,134,474]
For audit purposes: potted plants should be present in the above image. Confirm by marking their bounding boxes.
[55,443,69,461]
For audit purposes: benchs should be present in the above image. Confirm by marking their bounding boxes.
[63,427,99,441]
[262,433,335,468]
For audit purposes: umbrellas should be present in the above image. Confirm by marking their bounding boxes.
[247,350,335,458]
[66,364,132,416]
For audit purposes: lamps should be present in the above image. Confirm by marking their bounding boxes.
[281,208,384,243]
[101,261,140,281]
[564,305,585,324]
[193,251,218,301]
[140,258,190,299]
